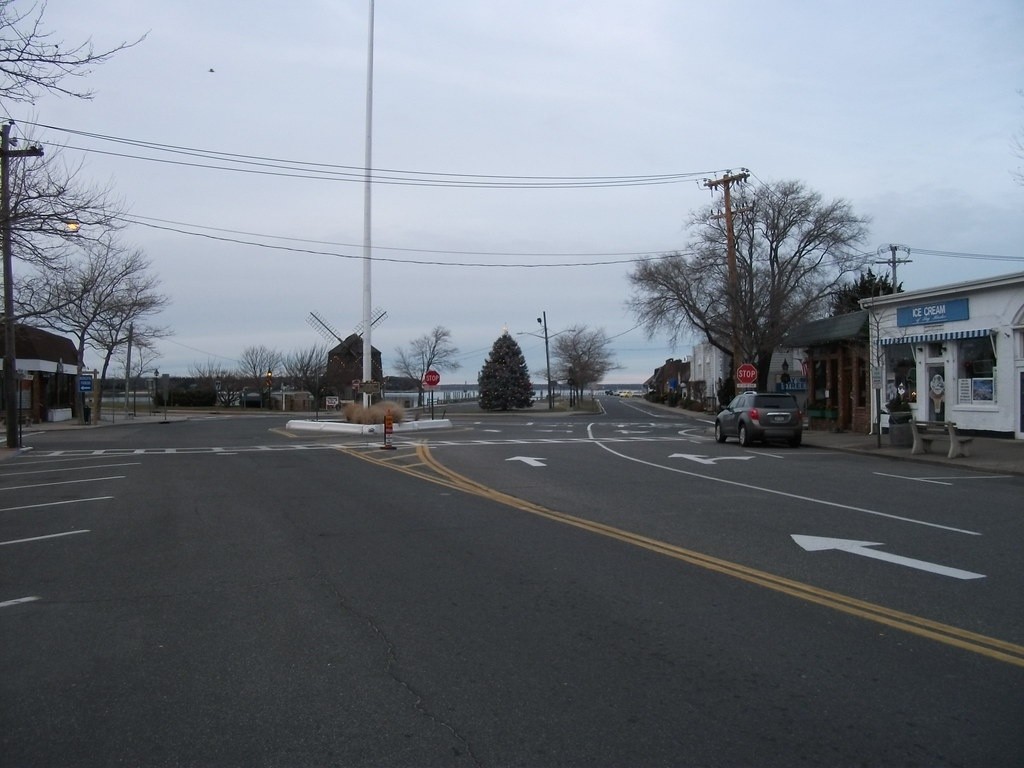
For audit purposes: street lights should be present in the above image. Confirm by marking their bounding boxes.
[297,369,318,422]
[516,318,576,409]
[2,215,79,449]
[154,369,159,409]
[266,366,272,408]
[782,357,789,389]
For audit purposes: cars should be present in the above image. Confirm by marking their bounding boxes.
[614,390,632,398]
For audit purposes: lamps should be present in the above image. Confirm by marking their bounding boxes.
[917,348,923,353]
[940,346,948,353]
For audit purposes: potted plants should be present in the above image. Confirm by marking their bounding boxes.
[885,398,916,448]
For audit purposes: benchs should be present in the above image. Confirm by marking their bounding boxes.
[908,420,974,458]
[17,417,34,427]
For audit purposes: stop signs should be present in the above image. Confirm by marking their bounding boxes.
[737,364,758,385]
[425,371,440,386]
[352,380,361,390]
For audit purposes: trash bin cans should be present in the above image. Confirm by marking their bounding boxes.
[83,405,91,425]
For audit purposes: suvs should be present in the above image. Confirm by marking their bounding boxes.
[714,390,803,446]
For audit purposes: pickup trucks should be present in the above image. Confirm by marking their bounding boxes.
[605,390,613,396]
